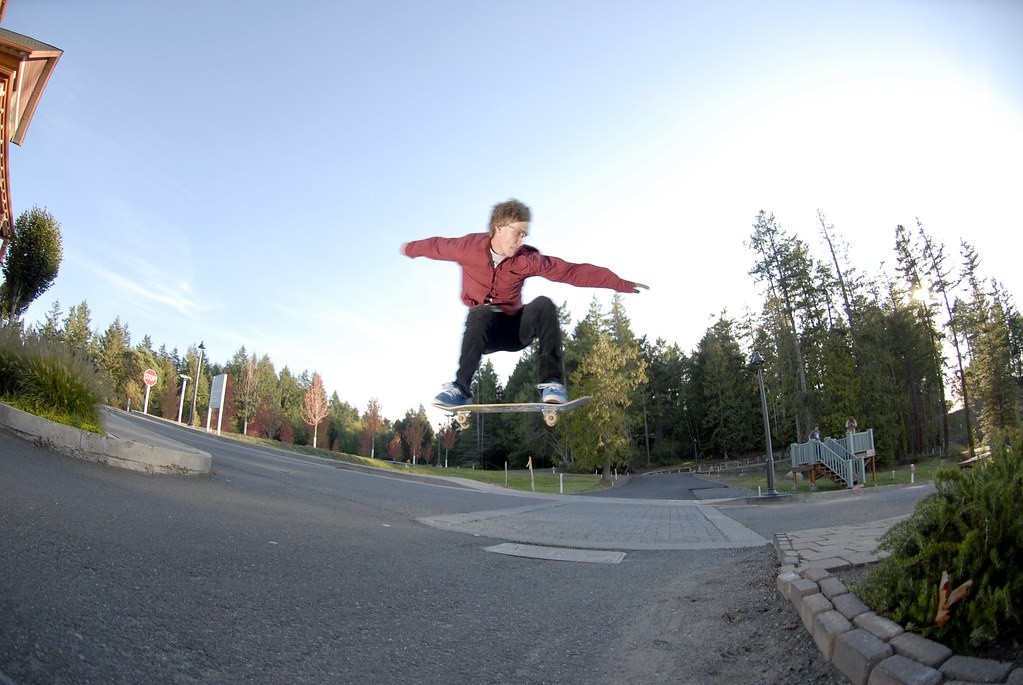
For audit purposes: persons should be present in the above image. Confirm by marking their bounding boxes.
[399,199,651,406]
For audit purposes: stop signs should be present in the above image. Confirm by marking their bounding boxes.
[143,369,158,387]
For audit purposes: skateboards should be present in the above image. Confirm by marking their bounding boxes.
[429,394,592,431]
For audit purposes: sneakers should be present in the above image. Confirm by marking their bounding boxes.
[537,381,568,404]
[433,382,472,407]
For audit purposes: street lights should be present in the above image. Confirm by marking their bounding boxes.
[187,340,206,425]
[750,352,778,496]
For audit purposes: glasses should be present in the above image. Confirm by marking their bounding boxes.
[507,223,528,238]
